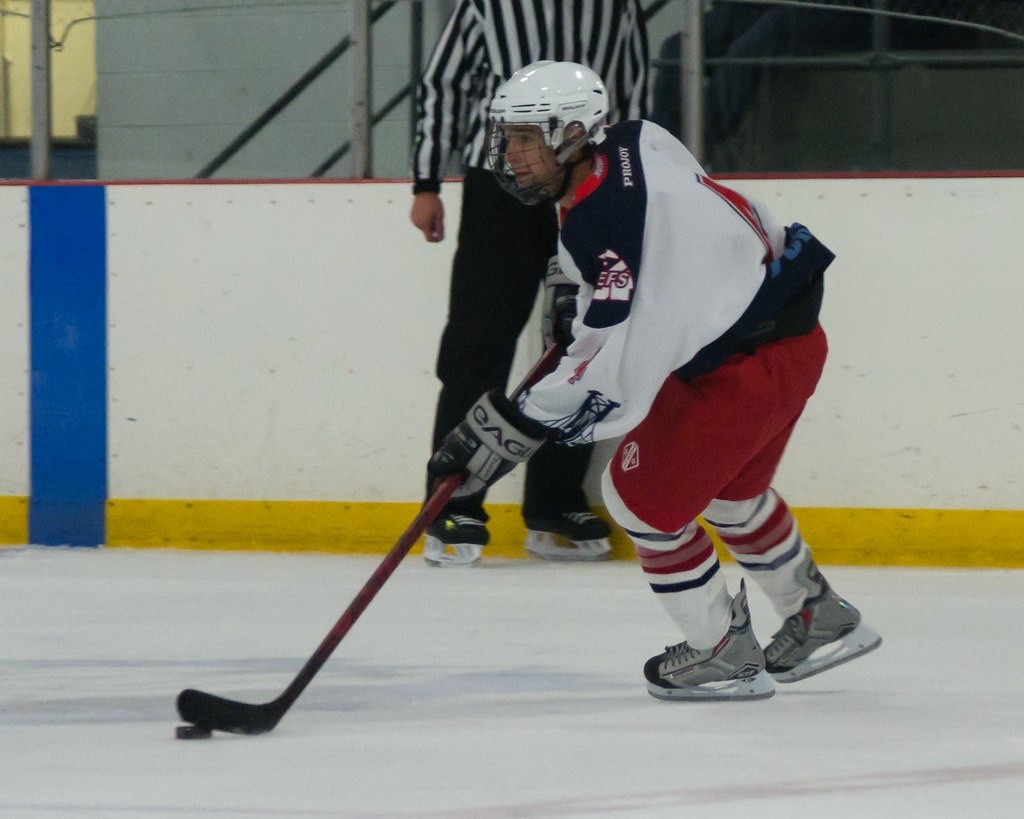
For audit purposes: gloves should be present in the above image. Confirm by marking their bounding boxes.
[429,383,553,499]
[537,252,588,348]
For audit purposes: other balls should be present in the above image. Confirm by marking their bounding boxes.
[174,725,211,739]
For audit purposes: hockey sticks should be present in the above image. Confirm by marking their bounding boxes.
[177,343,562,733]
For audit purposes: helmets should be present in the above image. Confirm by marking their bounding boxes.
[486,56,613,146]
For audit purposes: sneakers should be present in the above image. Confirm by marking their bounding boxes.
[760,591,888,689]
[644,621,778,699]
[520,497,615,562]
[420,504,492,573]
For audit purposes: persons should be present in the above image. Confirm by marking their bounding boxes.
[410,1,653,545]
[424,58,860,687]
